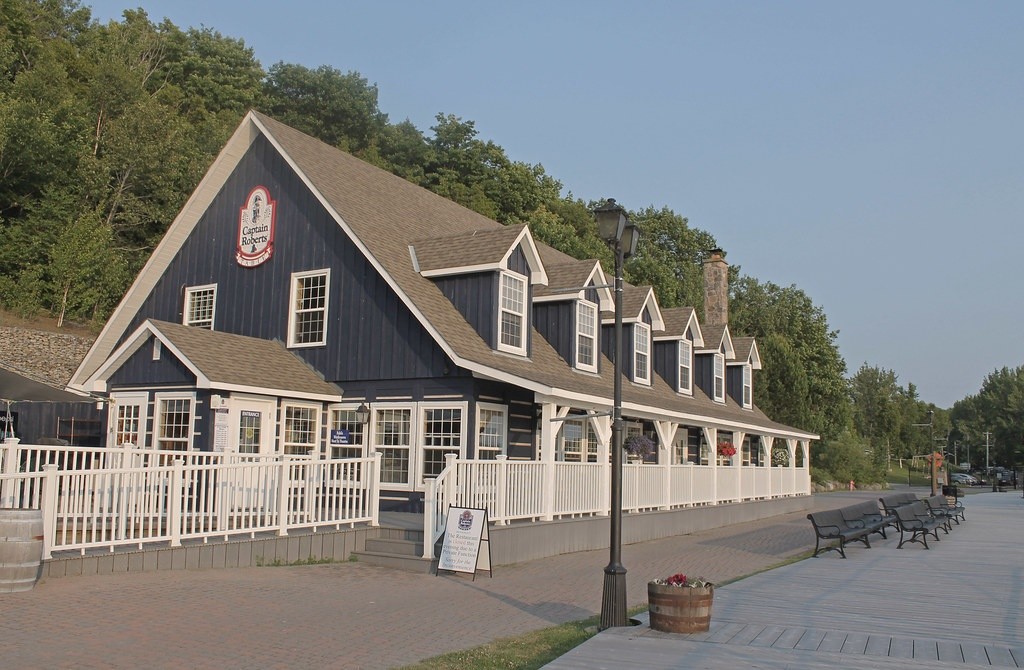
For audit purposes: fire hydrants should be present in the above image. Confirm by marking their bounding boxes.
[848,479,855,491]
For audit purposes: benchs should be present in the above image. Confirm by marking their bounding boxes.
[807,492,969,558]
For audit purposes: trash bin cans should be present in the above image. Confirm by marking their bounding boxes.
[942,485,957,504]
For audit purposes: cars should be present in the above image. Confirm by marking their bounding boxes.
[951,473,980,486]
[988,467,1018,486]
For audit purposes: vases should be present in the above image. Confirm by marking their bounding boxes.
[648,580,714,633]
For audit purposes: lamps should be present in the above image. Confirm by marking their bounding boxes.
[356,400,370,424]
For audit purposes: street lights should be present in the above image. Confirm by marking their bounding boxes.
[953,419,960,466]
[926,409,938,498]
[590,197,643,635]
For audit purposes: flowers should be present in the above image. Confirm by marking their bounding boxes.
[718,441,737,457]
[660,574,706,588]
[624,434,655,454]
[771,448,789,464]
